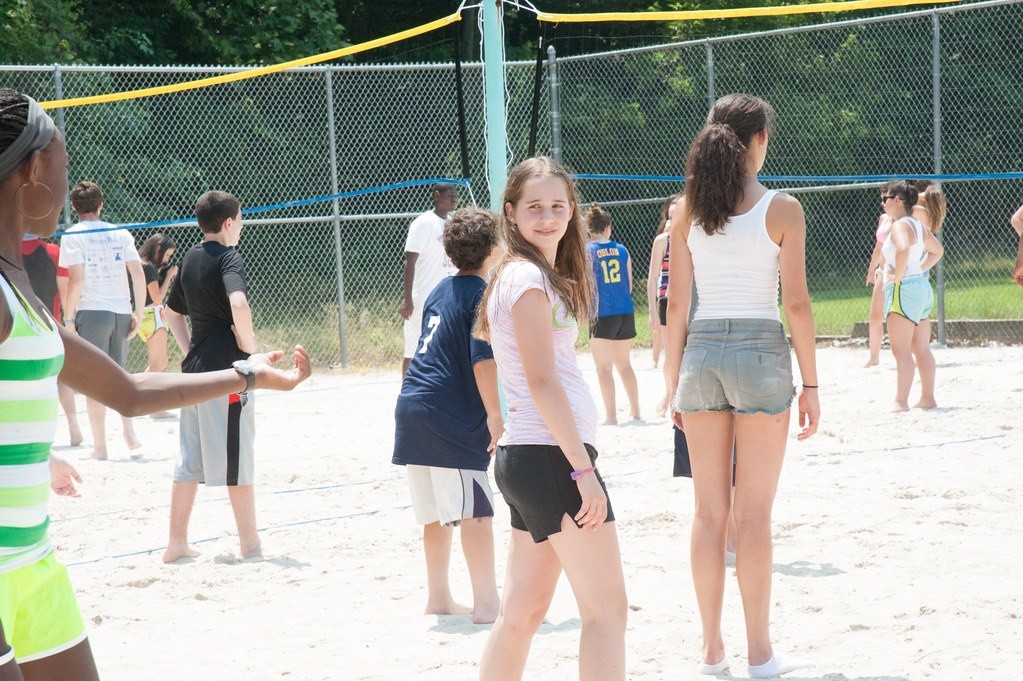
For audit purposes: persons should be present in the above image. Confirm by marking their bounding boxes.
[585,202,641,426]
[390,208,504,623]
[663,94,821,679]
[162,190,276,563]
[22,234,83,446]
[1011,205,1023,288]
[60,181,146,461]
[0,88,312,681]
[126,233,179,418]
[400,182,461,381]
[646,193,686,370]
[864,179,947,413]
[471,157,627,681]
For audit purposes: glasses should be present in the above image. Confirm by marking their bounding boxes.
[883,196,904,203]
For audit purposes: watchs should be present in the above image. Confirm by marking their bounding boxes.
[229,360,255,395]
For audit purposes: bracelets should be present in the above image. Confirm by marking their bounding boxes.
[570,467,596,480]
[63,318,75,322]
[803,384,819,388]
